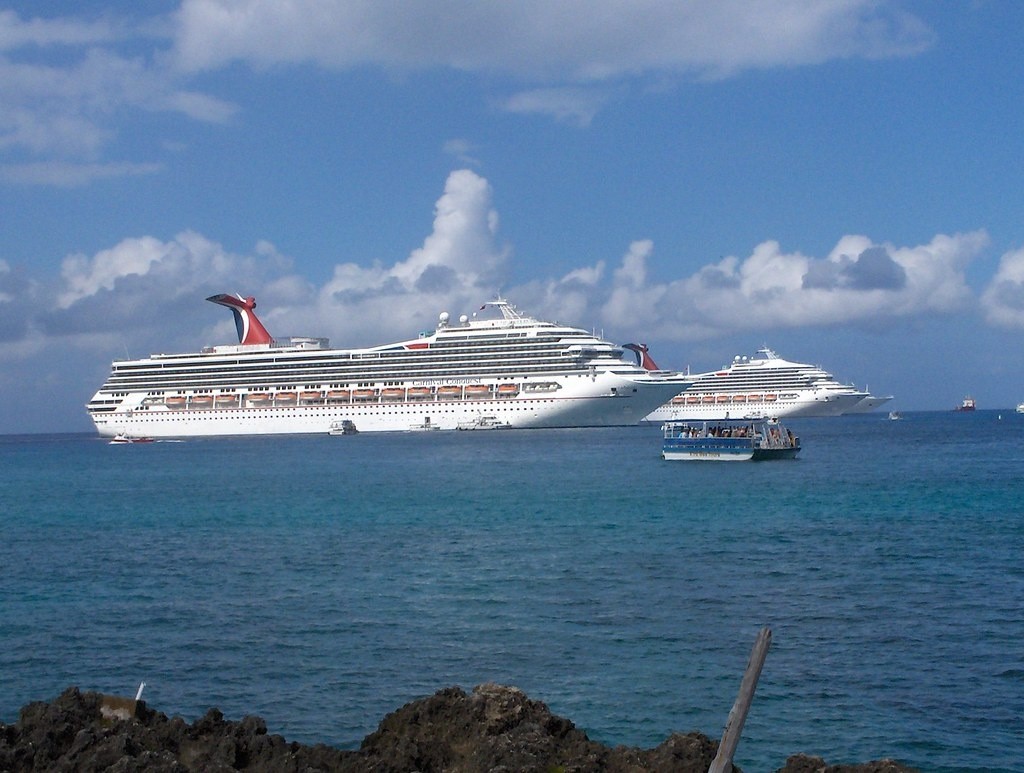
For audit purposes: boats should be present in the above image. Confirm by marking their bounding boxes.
[437,388,461,397]
[327,392,348,400]
[276,393,296,401]
[191,396,211,404]
[465,386,488,397]
[456,416,513,429]
[328,420,359,434]
[246,394,269,403]
[1015,403,1024,414]
[497,386,517,396]
[888,412,899,422]
[299,393,320,400]
[165,398,185,405]
[109,433,154,445]
[406,388,429,397]
[215,395,234,404]
[353,390,373,400]
[661,421,802,460]
[956,396,976,411]
[671,396,776,402]
[381,390,403,399]
[409,423,441,431]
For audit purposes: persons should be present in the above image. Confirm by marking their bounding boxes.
[679,426,754,437]
[769,428,795,447]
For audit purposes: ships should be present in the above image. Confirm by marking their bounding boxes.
[85,292,695,430]
[841,395,894,415]
[642,346,870,418]
